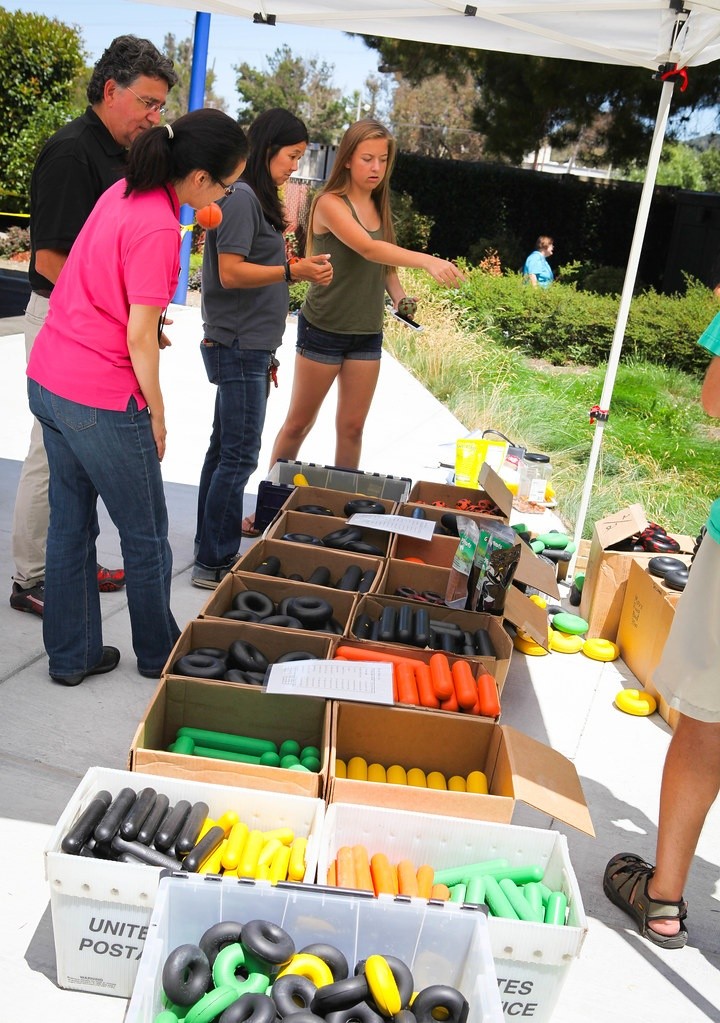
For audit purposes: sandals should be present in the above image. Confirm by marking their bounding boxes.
[603,853,690,949]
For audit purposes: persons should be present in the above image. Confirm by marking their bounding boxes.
[603,311,720,950]
[191,108,332,589]
[242,119,466,538]
[524,235,554,288]
[28,108,251,687]
[9,35,178,619]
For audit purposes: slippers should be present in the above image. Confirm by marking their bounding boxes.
[241,517,262,537]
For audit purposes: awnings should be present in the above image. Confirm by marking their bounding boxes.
[133,0,720,592]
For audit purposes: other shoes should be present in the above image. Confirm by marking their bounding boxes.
[192,579,221,590]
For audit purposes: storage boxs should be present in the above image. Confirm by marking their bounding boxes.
[331,702,597,843]
[266,507,392,558]
[334,639,502,724]
[395,500,505,539]
[231,537,384,601]
[374,558,552,656]
[162,615,332,687]
[348,594,515,696]
[388,531,561,602]
[333,801,596,1023]
[39,764,326,1008]
[407,471,513,526]
[124,869,505,1023]
[263,481,397,538]
[645,594,690,730]
[130,671,333,795]
[614,557,682,689]
[197,572,361,642]
[579,503,697,646]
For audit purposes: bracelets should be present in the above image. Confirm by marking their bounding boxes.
[284,263,293,284]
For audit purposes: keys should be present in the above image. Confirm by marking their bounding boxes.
[268,351,280,388]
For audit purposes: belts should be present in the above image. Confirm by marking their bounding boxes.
[32,289,51,299]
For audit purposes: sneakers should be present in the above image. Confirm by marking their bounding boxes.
[50,646,120,686]
[137,662,164,677]
[10,581,46,619]
[96,564,126,591]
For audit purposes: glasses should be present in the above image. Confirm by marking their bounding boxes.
[206,169,235,197]
[124,84,169,116]
